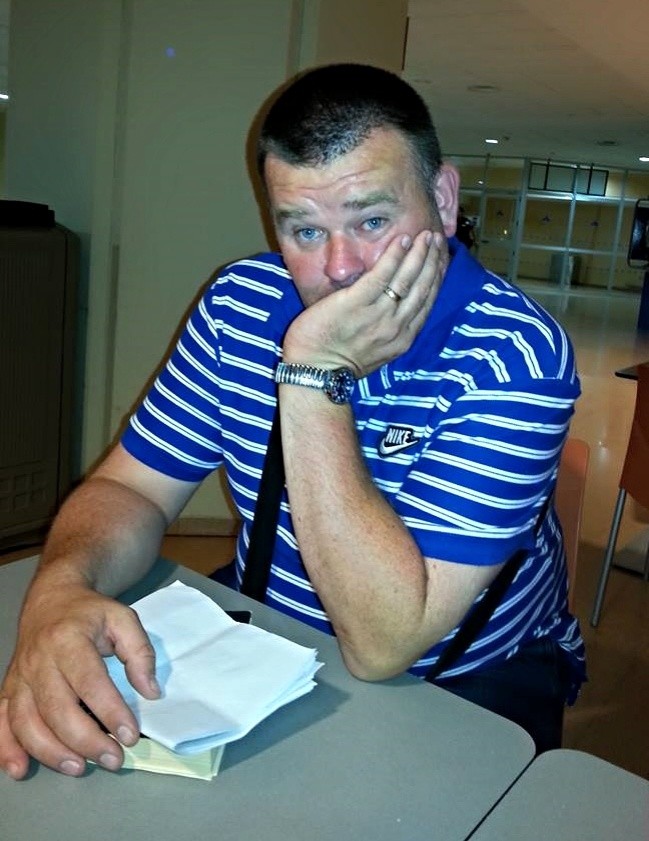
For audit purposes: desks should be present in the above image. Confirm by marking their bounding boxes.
[591,361,649,632]
[0,554,649,841]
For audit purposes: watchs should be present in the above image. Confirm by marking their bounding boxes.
[274,359,359,405]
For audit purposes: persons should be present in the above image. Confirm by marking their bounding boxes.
[2,63,591,784]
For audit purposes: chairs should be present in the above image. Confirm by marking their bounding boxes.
[551,436,589,614]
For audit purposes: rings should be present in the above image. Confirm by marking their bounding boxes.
[382,286,404,303]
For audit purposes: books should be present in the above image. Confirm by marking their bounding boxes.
[86,606,254,780]
